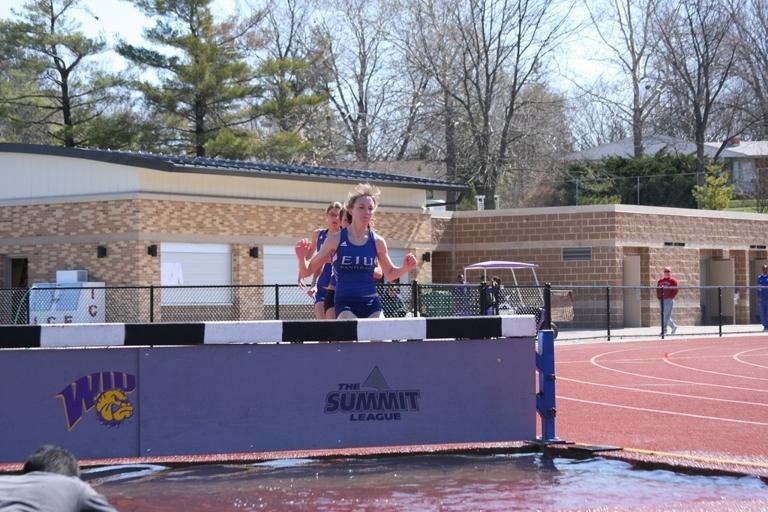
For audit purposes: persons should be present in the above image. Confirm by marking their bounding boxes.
[656,267,679,335]
[478,275,497,340]
[452,273,471,341]
[294,182,416,342]
[756,264,768,333]
[298,200,345,343]
[380,282,407,343]
[0,443,118,512]
[491,275,507,339]
[307,207,384,342]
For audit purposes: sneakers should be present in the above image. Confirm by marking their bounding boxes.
[671,325,677,334]
